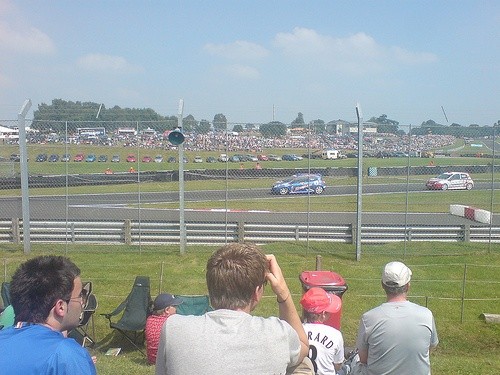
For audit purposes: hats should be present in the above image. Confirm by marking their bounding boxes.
[301,287,342,314]
[381,261,412,287]
[152,293,184,310]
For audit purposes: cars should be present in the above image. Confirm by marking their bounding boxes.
[126,154,136,162]
[47,154,60,162]
[111,154,120,162]
[229,147,425,163]
[98,155,108,162]
[61,153,72,162]
[193,156,203,163]
[425,172,475,191]
[35,153,47,162]
[153,156,164,163]
[167,157,176,163]
[206,156,217,163]
[85,154,97,162]
[10,153,30,162]
[74,153,84,162]
[142,155,153,162]
[183,157,188,163]
[271,172,327,195]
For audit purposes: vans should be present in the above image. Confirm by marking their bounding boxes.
[218,154,229,162]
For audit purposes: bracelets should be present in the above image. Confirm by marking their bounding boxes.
[277,291,290,303]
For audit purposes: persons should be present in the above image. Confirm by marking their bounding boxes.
[240,163,262,169]
[155,242,315,375]
[301,287,350,375]
[144,294,183,364]
[429,160,435,166]
[106,167,135,174]
[0,256,99,375]
[351,261,439,375]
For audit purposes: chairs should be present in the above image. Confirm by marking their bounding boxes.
[65,281,98,348]
[100,276,153,360]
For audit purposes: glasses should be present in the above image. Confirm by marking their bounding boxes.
[63,288,88,300]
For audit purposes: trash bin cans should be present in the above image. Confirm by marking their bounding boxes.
[299,269,348,330]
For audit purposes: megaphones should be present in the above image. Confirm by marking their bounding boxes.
[167,127,185,145]
